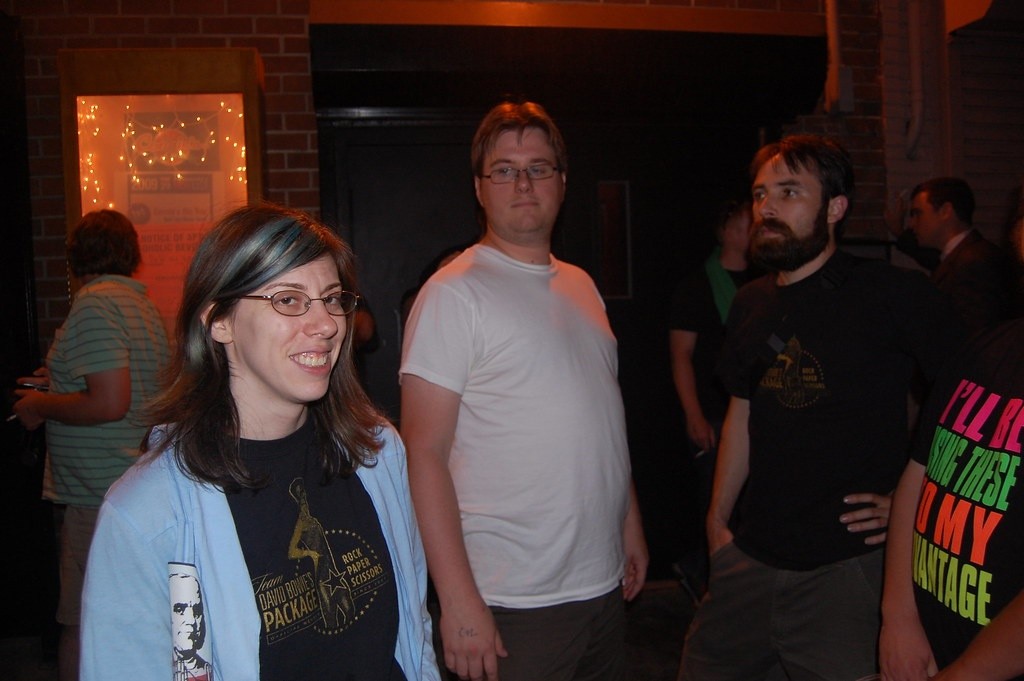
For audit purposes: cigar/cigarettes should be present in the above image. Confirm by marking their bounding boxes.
[695,450,706,457]
[6,415,17,422]
[900,188,909,197]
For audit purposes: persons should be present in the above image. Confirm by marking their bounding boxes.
[668,201,758,602]
[351,249,464,422]
[13,208,173,681]
[881,177,1024,459]
[874,189,1024,681]
[79,201,448,680]
[675,133,946,681]
[399,100,650,681]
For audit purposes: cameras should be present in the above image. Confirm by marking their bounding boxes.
[16,383,49,400]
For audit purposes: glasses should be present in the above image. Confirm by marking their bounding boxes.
[221,290,360,317]
[479,163,566,185]
